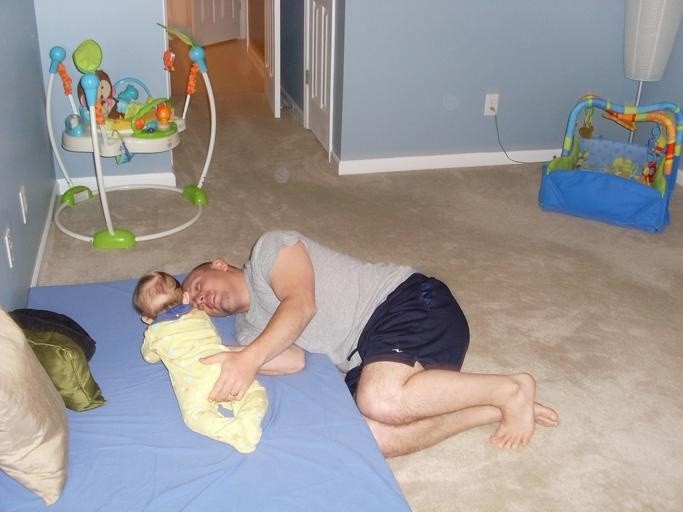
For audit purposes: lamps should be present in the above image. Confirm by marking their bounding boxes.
[625,0,683,143]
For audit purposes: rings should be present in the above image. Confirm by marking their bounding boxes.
[229,391,237,397]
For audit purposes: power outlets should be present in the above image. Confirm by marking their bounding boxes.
[484,94,498,116]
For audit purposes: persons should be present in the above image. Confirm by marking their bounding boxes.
[181,226,559,455]
[132,271,264,453]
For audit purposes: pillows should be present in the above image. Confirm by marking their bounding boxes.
[0,309,69,504]
[23,329,106,412]
[9,309,96,363]
